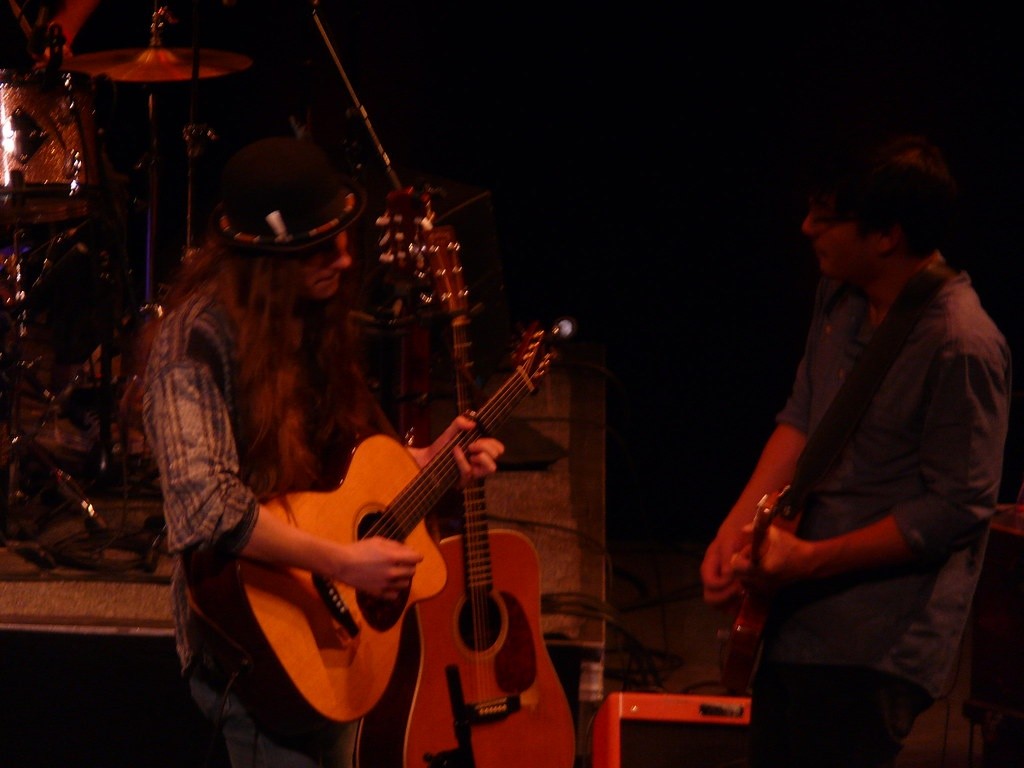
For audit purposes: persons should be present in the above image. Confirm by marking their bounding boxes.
[701,133,1011,768]
[135,136,507,768]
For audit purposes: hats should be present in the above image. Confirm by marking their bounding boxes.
[209,137,370,253]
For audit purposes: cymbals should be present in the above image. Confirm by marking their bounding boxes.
[56,42,253,82]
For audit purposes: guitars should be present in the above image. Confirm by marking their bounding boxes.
[353,223,576,767]
[721,481,798,696]
[188,315,578,748]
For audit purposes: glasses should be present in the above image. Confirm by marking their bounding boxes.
[808,198,855,228]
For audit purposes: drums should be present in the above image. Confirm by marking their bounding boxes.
[1,70,99,225]
